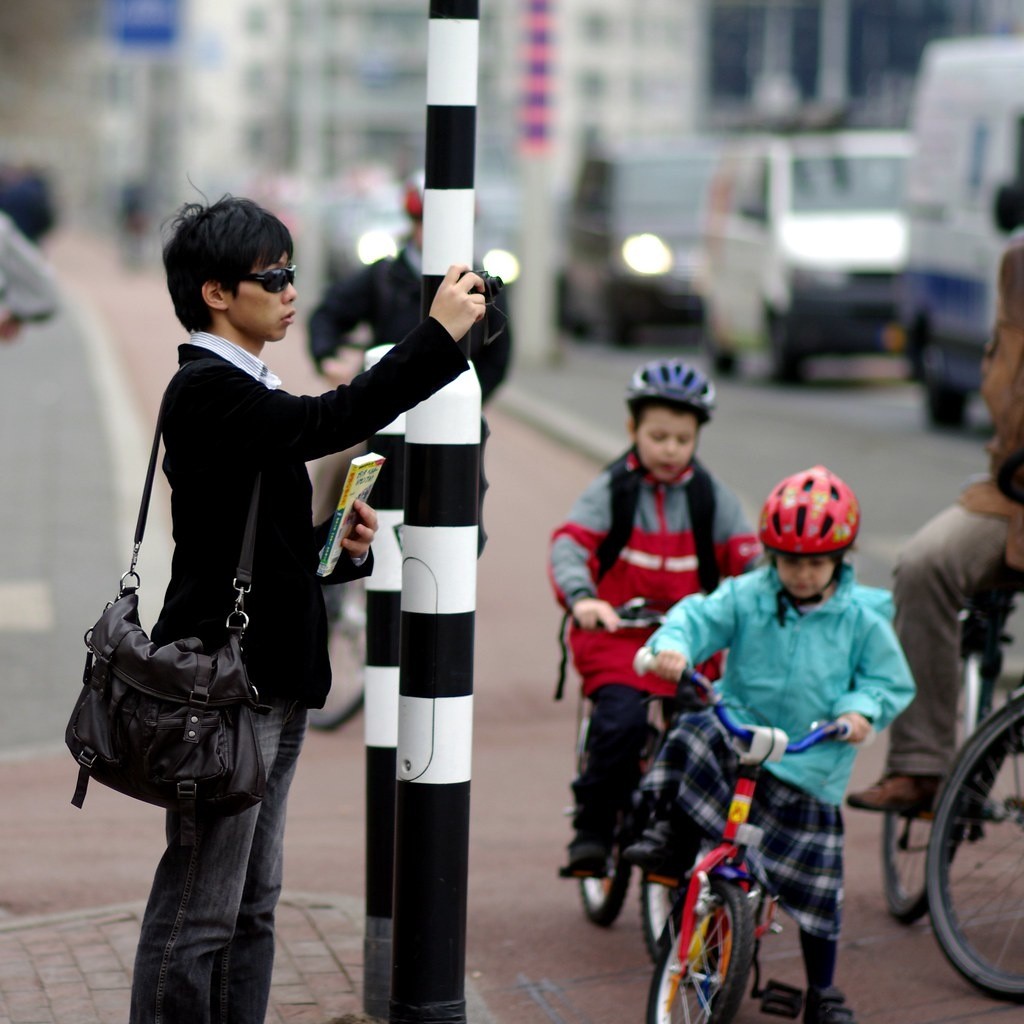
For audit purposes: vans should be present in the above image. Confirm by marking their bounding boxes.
[696,131,919,383]
[547,128,703,347]
[891,31,1023,425]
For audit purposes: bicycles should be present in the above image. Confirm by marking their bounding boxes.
[562,586,679,929]
[628,643,855,1023]
[884,565,1024,1007]
[308,584,378,736]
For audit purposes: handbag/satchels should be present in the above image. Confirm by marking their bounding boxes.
[64,586,266,816]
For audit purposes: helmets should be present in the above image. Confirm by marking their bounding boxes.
[404,165,479,222]
[625,357,716,408]
[759,465,860,551]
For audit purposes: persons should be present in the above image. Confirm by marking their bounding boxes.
[847,237,1024,822]
[126,197,488,1024]
[624,464,916,1024]
[311,168,513,623]
[548,355,768,878]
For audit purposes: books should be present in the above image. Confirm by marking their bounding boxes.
[315,453,385,577]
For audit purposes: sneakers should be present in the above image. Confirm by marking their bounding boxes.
[846,771,943,819]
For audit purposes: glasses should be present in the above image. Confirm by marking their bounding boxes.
[242,264,296,294]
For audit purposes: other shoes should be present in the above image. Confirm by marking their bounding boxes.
[801,985,858,1022]
[624,820,697,881]
[557,834,613,881]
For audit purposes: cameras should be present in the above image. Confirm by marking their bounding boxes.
[460,270,504,307]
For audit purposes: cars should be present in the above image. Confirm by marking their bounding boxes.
[972,180,1024,515]
